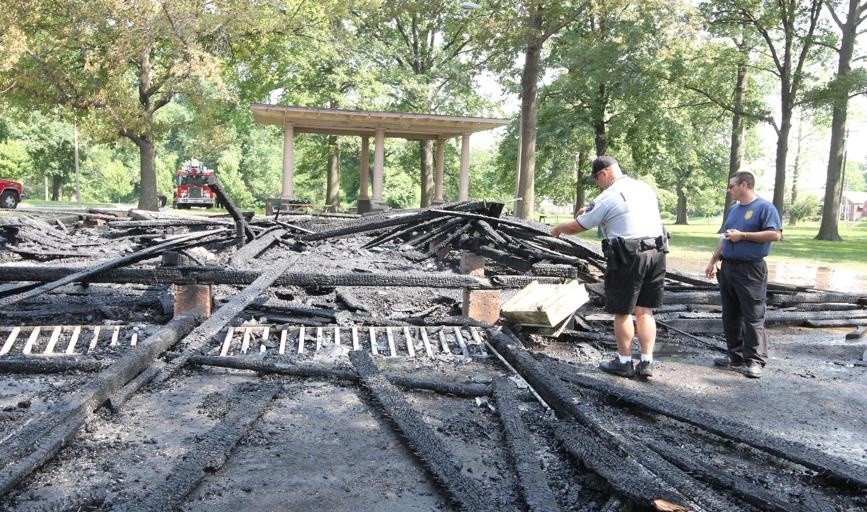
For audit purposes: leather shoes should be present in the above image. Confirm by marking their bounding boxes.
[599,357,634,377]
[745,361,762,377]
[635,360,652,376]
[714,356,745,366]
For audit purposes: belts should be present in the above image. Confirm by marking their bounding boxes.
[723,257,763,265]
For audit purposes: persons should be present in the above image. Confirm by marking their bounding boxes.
[551,156,669,377]
[705,172,782,377]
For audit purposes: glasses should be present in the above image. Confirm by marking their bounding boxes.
[593,172,604,180]
[728,184,735,189]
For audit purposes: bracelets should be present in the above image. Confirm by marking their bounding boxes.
[742,232,746,240]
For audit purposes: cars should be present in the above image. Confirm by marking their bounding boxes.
[0,178,24,210]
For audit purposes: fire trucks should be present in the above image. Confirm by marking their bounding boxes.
[172,157,216,210]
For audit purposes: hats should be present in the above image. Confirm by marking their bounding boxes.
[591,156,617,173]
